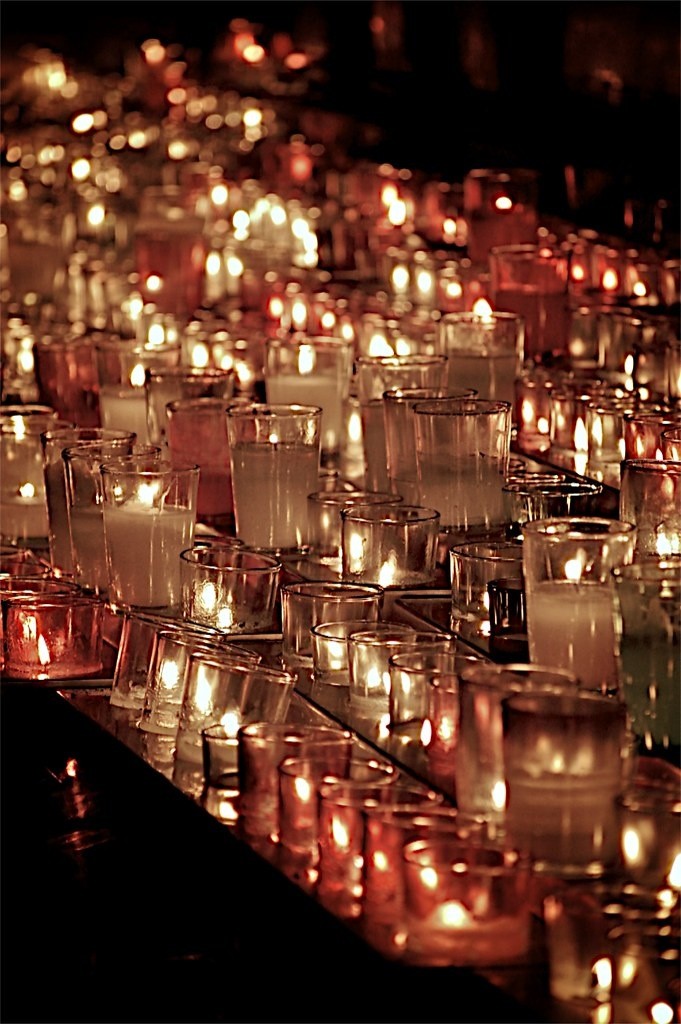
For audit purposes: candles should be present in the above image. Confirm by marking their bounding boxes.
[0,140,681,1024]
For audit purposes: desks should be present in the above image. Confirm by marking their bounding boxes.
[0,218,681,1024]
